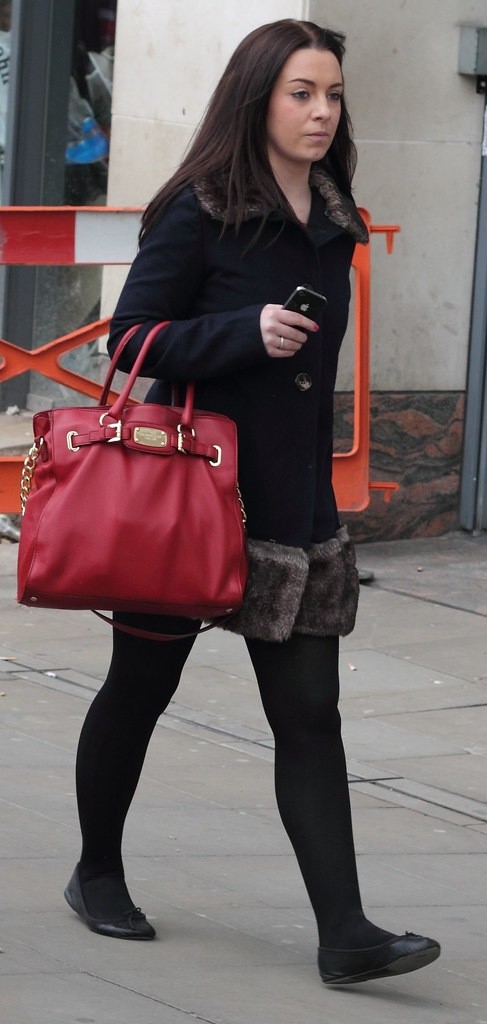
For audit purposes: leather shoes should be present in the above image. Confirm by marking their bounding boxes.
[63,862,156,940]
[318,931,441,984]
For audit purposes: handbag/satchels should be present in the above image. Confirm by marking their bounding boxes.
[17,321,248,642]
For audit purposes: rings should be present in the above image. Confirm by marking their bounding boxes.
[280,337,283,350]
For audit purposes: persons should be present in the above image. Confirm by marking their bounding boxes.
[63,19,441,984]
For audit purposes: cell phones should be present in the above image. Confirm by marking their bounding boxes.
[283,287,327,333]
